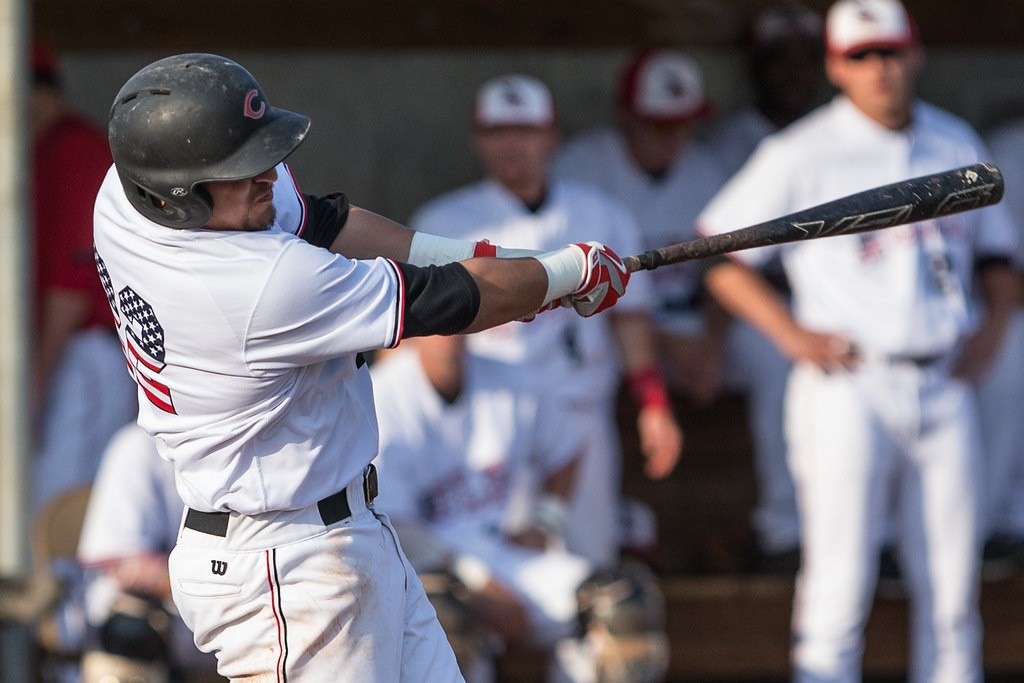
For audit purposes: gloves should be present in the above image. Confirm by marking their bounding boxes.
[473,240,573,323]
[560,241,632,319]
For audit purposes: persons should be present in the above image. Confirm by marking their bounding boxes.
[355,73,685,682]
[699,0,823,187]
[968,116,1024,556]
[548,45,802,574]
[91,53,630,683]
[695,0,1024,683]
[28,54,224,683]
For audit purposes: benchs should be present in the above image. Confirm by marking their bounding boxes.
[481,384,1023,683]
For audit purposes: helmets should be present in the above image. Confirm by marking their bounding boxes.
[109,52,312,232]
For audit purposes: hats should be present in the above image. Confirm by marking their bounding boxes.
[824,0,918,55]
[614,49,720,128]
[472,72,562,135]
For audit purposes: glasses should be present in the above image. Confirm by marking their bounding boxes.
[844,46,901,61]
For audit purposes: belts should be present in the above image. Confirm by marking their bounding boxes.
[184,464,379,540]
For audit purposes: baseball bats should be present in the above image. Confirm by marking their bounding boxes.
[620,160,1006,272]
